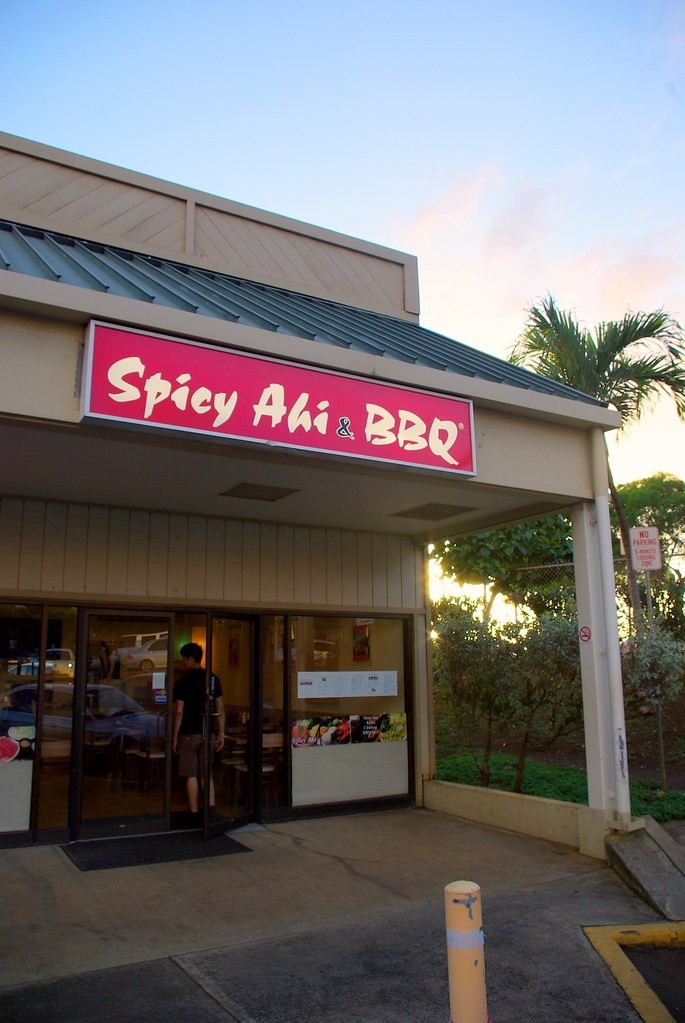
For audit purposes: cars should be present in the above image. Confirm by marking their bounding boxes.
[8,631,168,683]
[277,641,338,667]
[0,685,168,777]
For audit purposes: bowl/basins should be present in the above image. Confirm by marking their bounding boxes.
[291,721,319,747]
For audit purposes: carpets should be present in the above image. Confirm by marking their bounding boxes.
[60,829,253,872]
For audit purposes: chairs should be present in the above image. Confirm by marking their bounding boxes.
[121,712,284,809]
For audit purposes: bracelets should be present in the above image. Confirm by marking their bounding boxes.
[219,733,224,736]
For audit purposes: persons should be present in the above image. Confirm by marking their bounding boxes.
[0,683,37,753]
[354,630,368,656]
[171,642,226,813]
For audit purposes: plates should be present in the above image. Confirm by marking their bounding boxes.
[0,736,20,762]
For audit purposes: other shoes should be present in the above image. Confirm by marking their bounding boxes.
[209,805,223,820]
